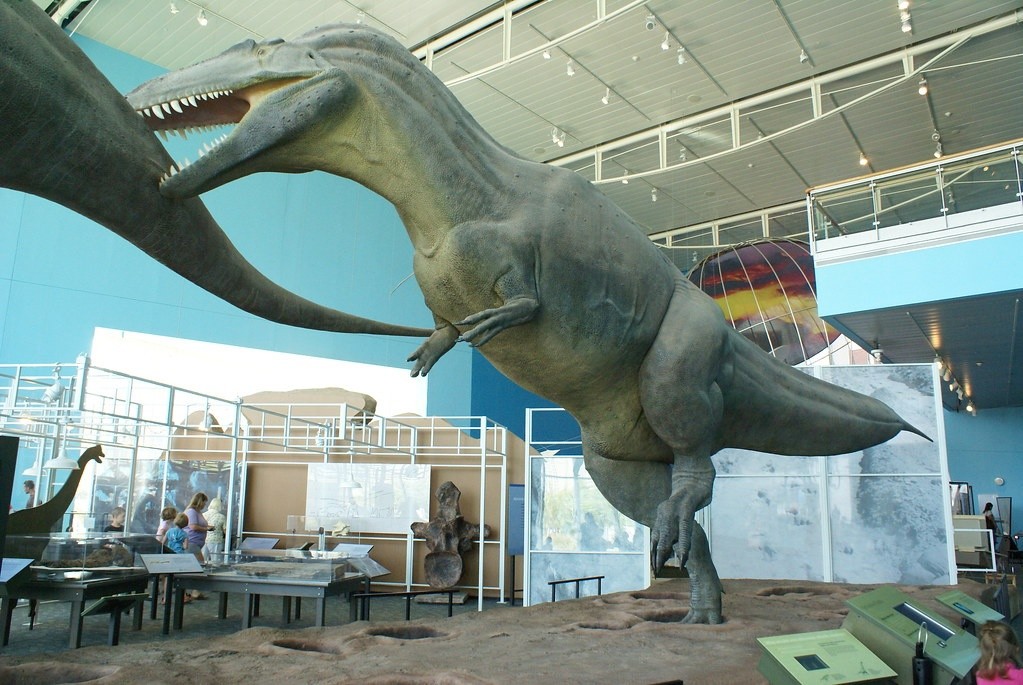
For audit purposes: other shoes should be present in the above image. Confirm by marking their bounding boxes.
[161,597,165,605]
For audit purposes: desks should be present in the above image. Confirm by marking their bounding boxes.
[167,566,369,634]
[0,566,154,650]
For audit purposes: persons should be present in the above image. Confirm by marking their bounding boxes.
[24,481,42,508]
[983,503,998,551]
[99,507,126,550]
[976,620,1023,685]
[233,498,240,548]
[202,498,226,561]
[155,507,194,605]
[183,492,216,600]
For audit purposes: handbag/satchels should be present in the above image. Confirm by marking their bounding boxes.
[986,515,996,529]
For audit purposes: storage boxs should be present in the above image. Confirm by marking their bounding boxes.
[3,532,163,586]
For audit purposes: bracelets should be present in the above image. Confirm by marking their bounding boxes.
[207,527,210,531]
[110,544,112,548]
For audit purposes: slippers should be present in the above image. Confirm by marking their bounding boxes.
[191,593,208,600]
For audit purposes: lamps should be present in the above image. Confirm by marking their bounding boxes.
[972,406,978,417]
[934,142,945,159]
[645,13,657,30]
[932,353,944,368]
[917,76,929,95]
[650,187,659,202]
[858,151,868,166]
[542,40,554,60]
[170,1,180,14]
[940,363,947,376]
[550,127,568,148]
[931,128,942,143]
[870,343,886,363]
[197,9,208,26]
[567,58,577,77]
[757,131,762,139]
[679,145,688,161]
[622,168,631,185]
[356,11,366,24]
[800,47,809,64]
[966,400,974,412]
[944,367,952,382]
[661,31,672,51]
[946,191,955,205]
[601,87,612,106]
[948,378,960,391]
[677,46,687,66]
[956,388,966,400]
